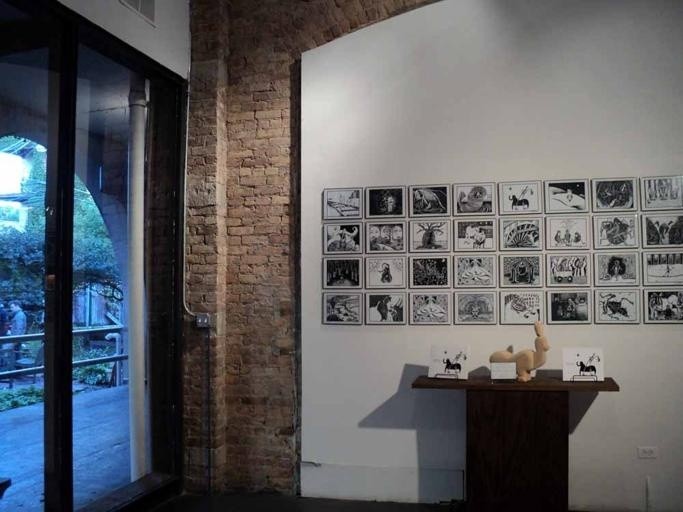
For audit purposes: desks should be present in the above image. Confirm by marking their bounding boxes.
[410,374,619,511]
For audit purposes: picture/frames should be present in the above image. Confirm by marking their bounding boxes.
[319,175,683,325]
